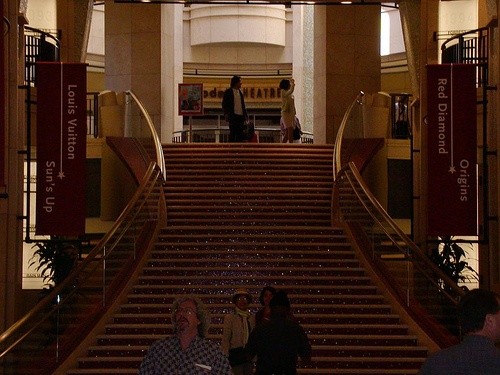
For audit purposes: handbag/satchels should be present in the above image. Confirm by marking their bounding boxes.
[243,121,254,141]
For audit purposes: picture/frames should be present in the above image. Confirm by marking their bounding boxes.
[178,83,205,115]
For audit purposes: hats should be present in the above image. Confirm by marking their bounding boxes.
[236,287,249,295]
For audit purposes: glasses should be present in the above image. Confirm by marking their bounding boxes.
[238,297,247,301]
[174,307,193,315]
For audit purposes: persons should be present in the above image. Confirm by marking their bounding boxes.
[419,289,500,375]
[254,286,275,334]
[220,288,255,375]
[135,294,233,375]
[278,78,296,143]
[222,75,249,142]
[244,293,311,375]
